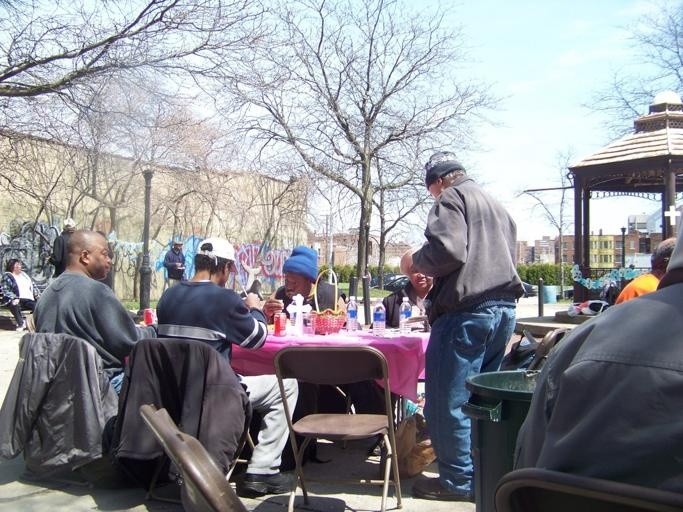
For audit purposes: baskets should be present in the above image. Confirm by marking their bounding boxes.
[307,307,347,336]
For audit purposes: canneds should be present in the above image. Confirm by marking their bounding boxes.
[272,313,288,335]
[142,309,155,325]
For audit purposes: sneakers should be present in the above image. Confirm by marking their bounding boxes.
[412,479,473,500]
[236,471,296,497]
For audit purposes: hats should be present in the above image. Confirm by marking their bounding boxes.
[172,239,183,245]
[196,238,238,273]
[282,245,319,283]
[424,151,465,191]
[62,218,77,231]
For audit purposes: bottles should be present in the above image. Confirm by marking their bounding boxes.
[399,297,412,334]
[289,295,295,336]
[373,298,386,337]
[346,295,357,334]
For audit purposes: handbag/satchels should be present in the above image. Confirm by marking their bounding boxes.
[499,329,546,373]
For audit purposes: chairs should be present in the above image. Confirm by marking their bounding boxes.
[18,332,139,498]
[274,346,403,512]
[139,404,248,512]
[492,467,683,511]
[527,327,573,372]
[121,339,255,506]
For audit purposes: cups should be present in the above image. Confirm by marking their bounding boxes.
[361,324,369,334]
[274,300,284,313]
[303,314,316,337]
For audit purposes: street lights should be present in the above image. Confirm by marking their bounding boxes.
[133,162,154,322]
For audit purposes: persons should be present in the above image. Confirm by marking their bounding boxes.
[411,152,526,502]
[615,237,675,305]
[368,246,434,454]
[261,245,349,414]
[512,213,683,495]
[0,258,39,330]
[94,231,113,290]
[163,241,186,287]
[157,238,295,495]
[46,218,76,277]
[34,229,157,396]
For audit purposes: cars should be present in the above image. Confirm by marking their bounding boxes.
[383,277,409,291]
[522,282,537,298]
